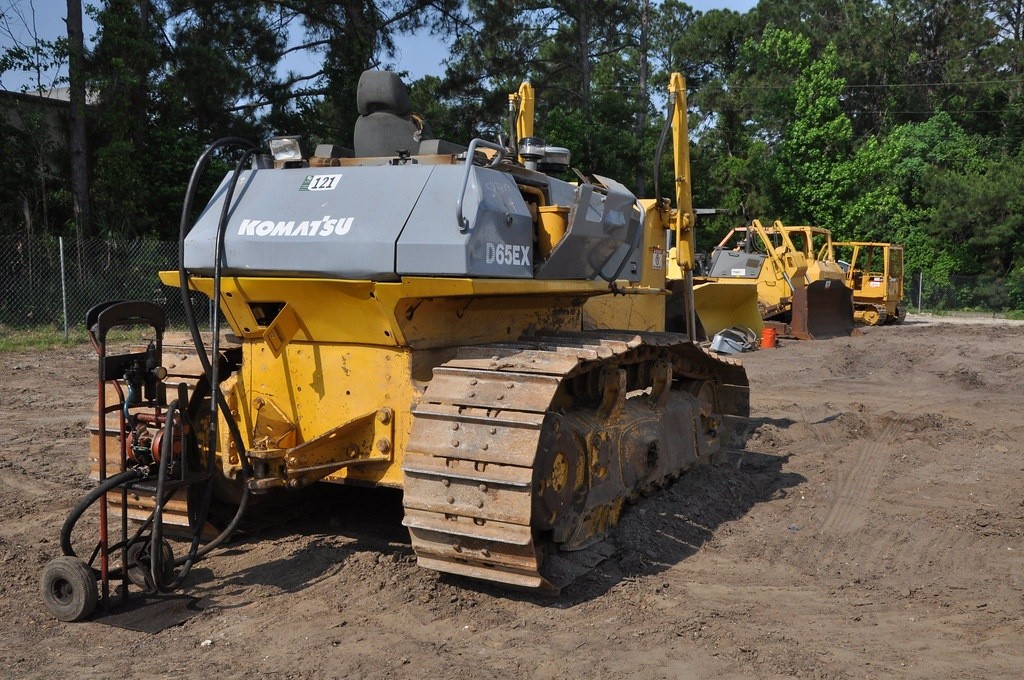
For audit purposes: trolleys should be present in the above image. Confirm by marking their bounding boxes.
[47,299,214,633]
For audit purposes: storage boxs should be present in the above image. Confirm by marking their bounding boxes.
[710,328,749,355]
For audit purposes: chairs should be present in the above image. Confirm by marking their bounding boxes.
[354,70,436,158]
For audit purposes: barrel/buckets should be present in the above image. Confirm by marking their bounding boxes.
[760,327,776,349]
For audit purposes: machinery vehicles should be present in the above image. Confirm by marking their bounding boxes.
[71,71,903,604]
